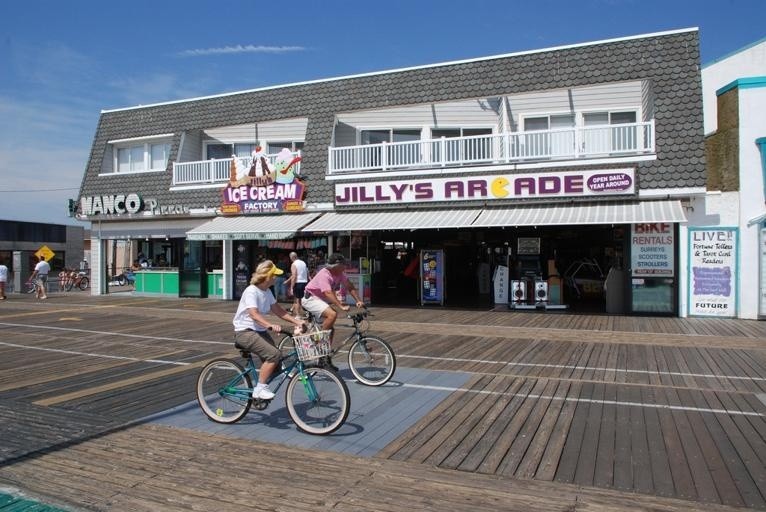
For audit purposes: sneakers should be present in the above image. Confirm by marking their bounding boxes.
[318,355,339,371]
[252,385,276,400]
[285,308,302,320]
[40,294,49,299]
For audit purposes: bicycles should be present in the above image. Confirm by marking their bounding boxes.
[119,268,135,286]
[196,323,351,436]
[277,305,396,387]
[64,270,89,292]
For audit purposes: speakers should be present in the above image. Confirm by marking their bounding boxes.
[534,280,548,301]
[511,279,527,301]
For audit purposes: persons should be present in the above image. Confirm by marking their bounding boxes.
[232,259,309,401]
[300,253,365,373]
[30,256,52,300]
[0,259,8,300]
[58,268,69,288]
[124,251,326,319]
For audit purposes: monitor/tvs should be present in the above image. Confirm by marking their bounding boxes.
[518,237,541,255]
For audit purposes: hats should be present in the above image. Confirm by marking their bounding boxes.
[327,252,352,265]
[256,262,285,278]
